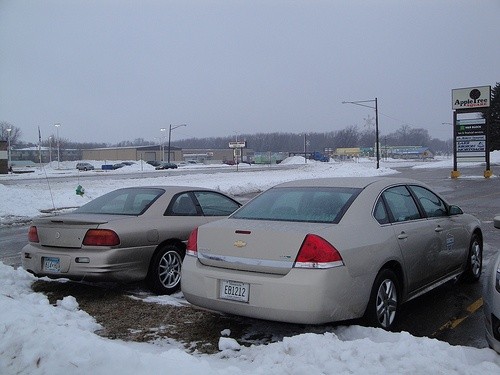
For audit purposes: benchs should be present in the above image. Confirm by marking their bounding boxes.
[273,206,298,215]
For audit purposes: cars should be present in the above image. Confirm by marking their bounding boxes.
[178,177,484,330]
[484,215,500,350]
[155,162,178,170]
[221,159,256,164]
[148,160,162,166]
[20,185,246,294]
[111,164,121,170]
[189,160,201,164]
[177,162,188,167]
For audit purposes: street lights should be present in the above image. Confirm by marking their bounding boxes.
[161,128,171,164]
[6,128,13,173]
[343,98,385,170]
[168,124,192,168]
[54,123,63,168]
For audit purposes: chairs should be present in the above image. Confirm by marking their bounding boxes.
[178,197,194,214]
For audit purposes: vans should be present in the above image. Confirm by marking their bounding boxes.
[76,163,95,171]
